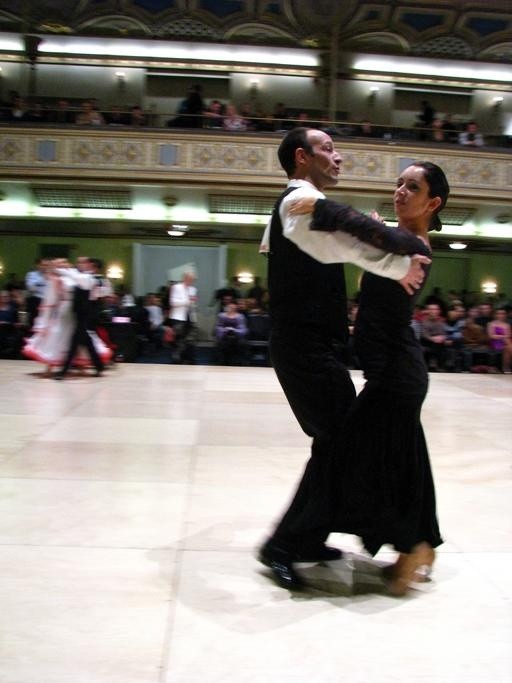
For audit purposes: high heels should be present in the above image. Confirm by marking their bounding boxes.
[45,366,105,380]
[381,543,437,597]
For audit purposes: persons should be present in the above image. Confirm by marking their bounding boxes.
[289,160,450,597]
[0,253,511,382]
[2,84,484,149]
[259,128,432,590]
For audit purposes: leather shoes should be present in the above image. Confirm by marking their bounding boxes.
[292,542,342,562]
[257,545,306,591]
